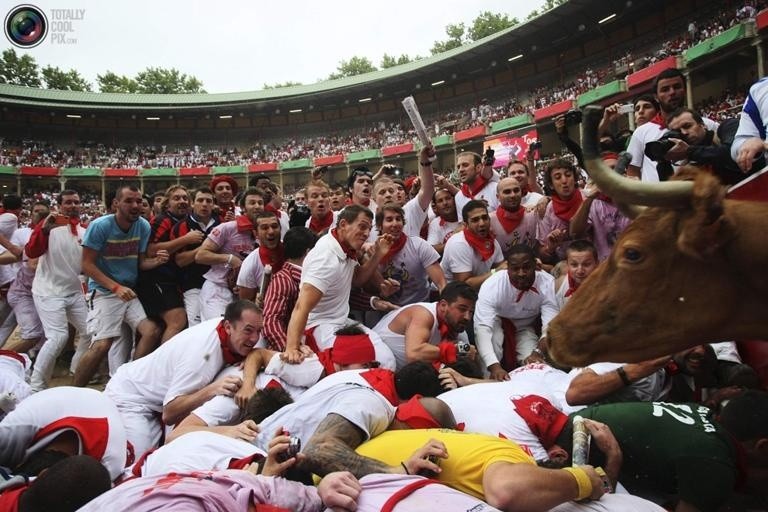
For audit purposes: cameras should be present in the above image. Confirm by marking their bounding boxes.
[485,149,495,166]
[644,132,683,159]
[276,430,302,463]
[423,455,441,478]
[63,210,80,221]
[560,109,581,124]
[295,203,309,215]
[382,167,406,178]
[616,104,634,114]
[457,341,477,355]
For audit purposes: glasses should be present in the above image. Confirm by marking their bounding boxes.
[352,170,374,176]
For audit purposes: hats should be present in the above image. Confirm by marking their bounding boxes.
[210,176,238,198]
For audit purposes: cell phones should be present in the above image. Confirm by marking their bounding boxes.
[321,165,329,173]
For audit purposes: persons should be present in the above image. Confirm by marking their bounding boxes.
[0,1,768,512]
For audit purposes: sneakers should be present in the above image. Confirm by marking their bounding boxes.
[68,369,101,383]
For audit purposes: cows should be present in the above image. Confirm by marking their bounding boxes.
[541,102,768,371]
